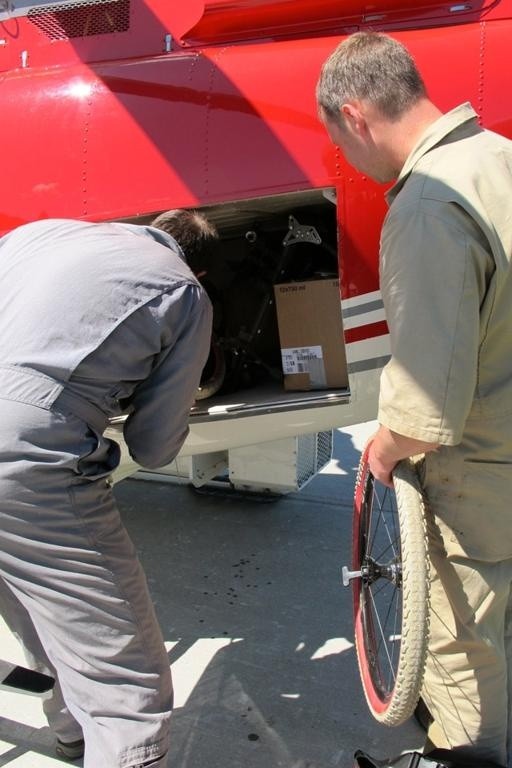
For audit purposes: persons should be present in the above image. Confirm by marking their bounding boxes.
[2,210,224,768]
[314,30,511,768]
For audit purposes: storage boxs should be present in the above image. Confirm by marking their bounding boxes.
[269,269,350,396]
